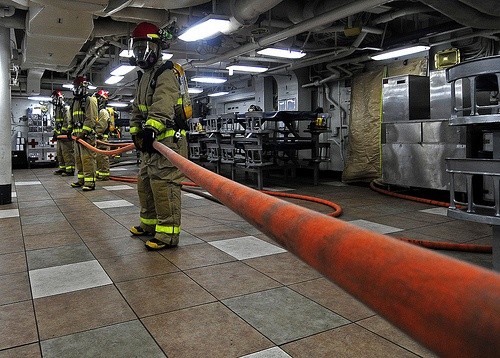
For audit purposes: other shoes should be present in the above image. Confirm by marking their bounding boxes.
[62,172,75,176]
[54,168,66,175]
[144,238,178,251]
[96,175,109,181]
[82,182,95,191]
[70,182,83,187]
[129,225,156,237]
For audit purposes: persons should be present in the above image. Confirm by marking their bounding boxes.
[50,90,75,176]
[129,21,189,250]
[67,76,99,191]
[95,90,111,181]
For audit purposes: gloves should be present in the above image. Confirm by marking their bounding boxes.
[141,128,155,153]
[53,134,58,141]
[67,130,74,142]
[76,133,88,144]
[130,134,142,151]
[57,133,64,140]
[97,135,103,140]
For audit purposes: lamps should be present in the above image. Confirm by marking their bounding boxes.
[257,40,306,58]
[226,59,269,73]
[174,0,230,42]
[207,84,229,97]
[187,82,203,93]
[371,44,431,61]
[191,69,227,83]
[104,37,137,84]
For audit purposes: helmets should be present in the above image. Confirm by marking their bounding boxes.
[131,22,160,39]
[51,91,63,98]
[96,89,109,100]
[72,76,89,89]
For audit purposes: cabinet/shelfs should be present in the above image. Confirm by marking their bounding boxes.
[185,110,332,190]
[26,114,58,165]
